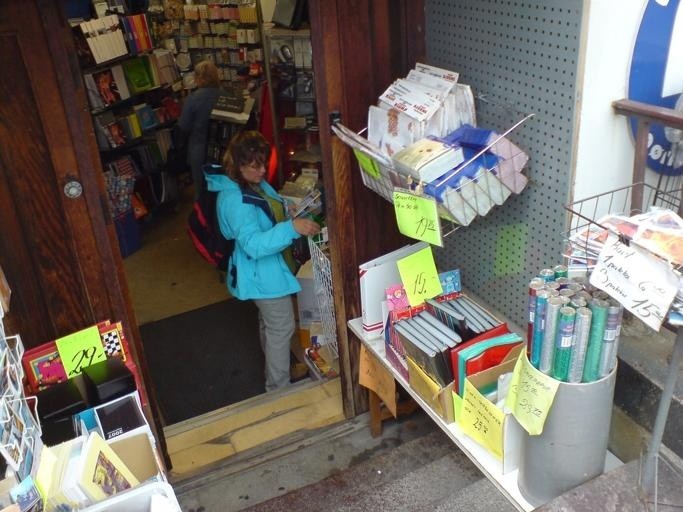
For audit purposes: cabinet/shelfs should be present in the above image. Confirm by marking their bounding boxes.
[68,1,193,221]
[142,1,263,164]
[255,1,325,190]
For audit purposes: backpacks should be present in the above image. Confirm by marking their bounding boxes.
[184,180,236,270]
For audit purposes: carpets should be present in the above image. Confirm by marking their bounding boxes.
[139,295,309,425]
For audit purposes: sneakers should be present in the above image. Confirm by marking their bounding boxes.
[290,364,309,379]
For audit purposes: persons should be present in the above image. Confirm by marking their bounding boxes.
[212,131,324,391]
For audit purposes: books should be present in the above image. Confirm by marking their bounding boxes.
[332,56,533,229]
[559,206,682,329]
[0,300,154,511]
[382,290,524,388]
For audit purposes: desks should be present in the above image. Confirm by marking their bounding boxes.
[347,314,624,512]
